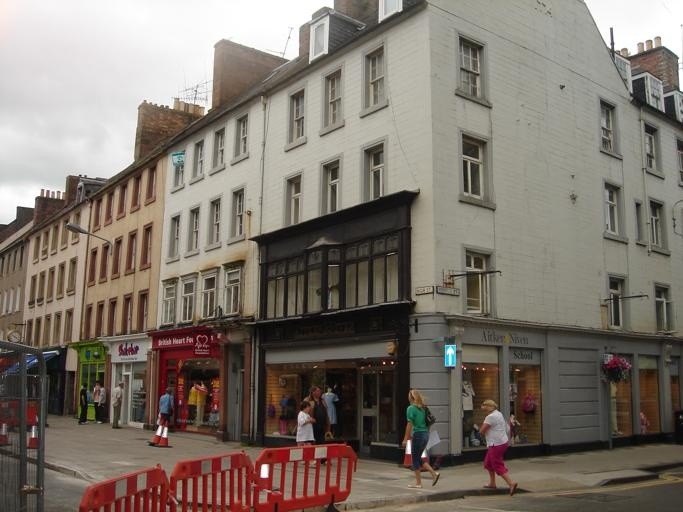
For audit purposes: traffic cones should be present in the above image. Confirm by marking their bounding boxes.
[147,418,170,449]
[0,420,10,447]
[420,443,431,471]
[401,435,414,468]
[28,421,39,450]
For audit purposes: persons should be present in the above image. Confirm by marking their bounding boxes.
[159,387,175,425]
[278,394,289,435]
[479,399,518,496]
[92,381,106,424]
[509,413,520,443]
[187,384,198,420]
[111,382,124,429]
[295,401,316,446]
[303,385,331,464]
[78,382,91,425]
[321,387,339,425]
[197,383,207,424]
[402,390,440,488]
[462,380,475,445]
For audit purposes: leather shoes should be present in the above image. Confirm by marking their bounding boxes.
[407,484,423,488]
[432,472,440,486]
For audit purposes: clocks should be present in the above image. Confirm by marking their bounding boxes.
[6,330,22,344]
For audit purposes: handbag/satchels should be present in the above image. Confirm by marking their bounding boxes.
[167,394,174,417]
[424,407,436,427]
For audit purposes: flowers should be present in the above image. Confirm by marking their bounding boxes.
[603,358,631,383]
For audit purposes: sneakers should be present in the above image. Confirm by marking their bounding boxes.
[78,421,104,424]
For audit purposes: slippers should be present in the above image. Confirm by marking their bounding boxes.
[484,483,496,489]
[510,482,518,496]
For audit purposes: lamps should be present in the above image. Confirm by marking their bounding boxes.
[64,220,112,254]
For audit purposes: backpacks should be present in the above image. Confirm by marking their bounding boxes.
[520,392,536,413]
